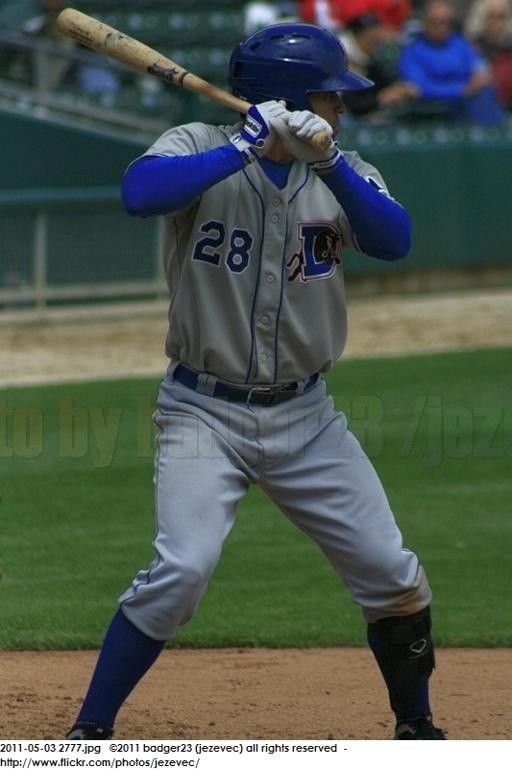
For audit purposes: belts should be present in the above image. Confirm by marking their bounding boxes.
[174,366,322,405]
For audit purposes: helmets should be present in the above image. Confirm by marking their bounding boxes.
[229,23,375,105]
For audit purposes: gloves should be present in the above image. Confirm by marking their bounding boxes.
[269,108,341,169]
[229,99,291,162]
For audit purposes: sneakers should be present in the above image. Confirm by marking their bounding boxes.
[395,718,446,739]
[66,721,114,739]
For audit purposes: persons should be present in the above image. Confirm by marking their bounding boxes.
[66,22,446,739]
[297,0,512,130]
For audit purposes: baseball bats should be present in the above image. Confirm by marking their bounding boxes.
[55,4,333,151]
